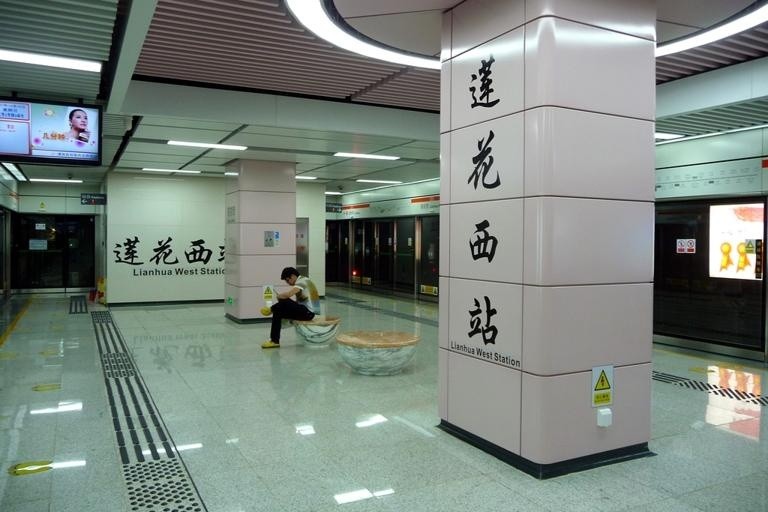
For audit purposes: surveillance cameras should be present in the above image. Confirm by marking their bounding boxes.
[68,175,73,179]
[339,185,344,190]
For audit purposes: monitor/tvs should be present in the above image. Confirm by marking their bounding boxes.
[0,95,103,168]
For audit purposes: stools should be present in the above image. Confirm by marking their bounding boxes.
[291,315,342,349]
[335,331,420,376]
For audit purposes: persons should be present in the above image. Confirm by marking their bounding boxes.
[260,267,320,347]
[62,109,88,143]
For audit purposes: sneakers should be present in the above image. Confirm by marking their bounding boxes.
[261,340,280,348]
[260,306,272,316]
